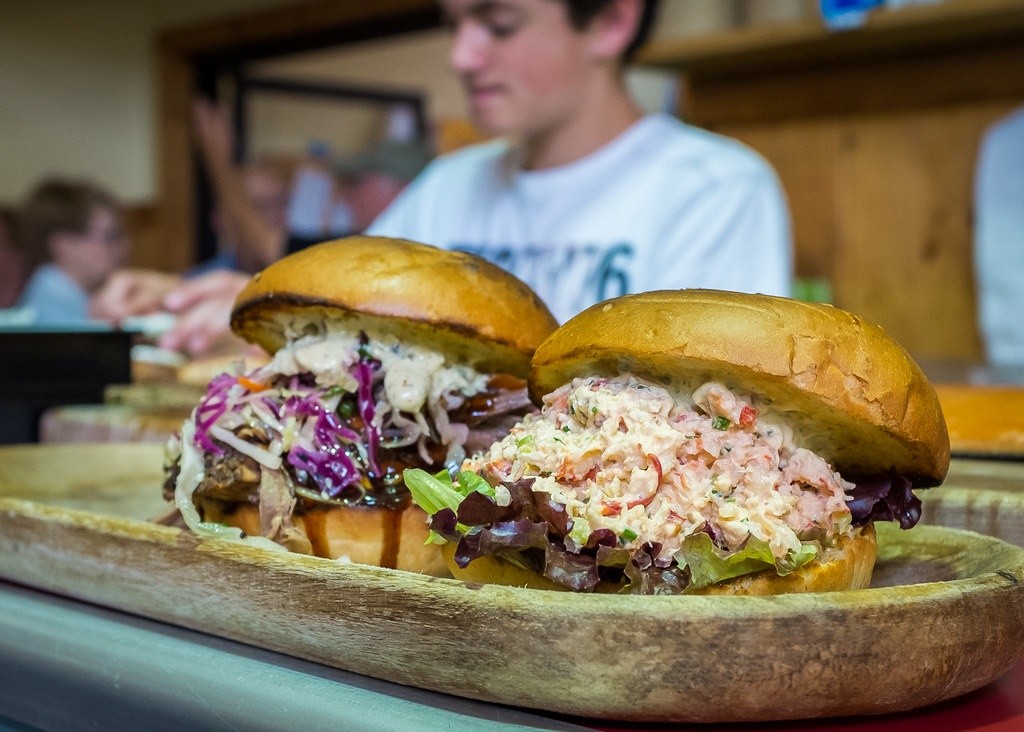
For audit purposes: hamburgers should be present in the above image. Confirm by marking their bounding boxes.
[403,286,952,597]
[160,234,561,576]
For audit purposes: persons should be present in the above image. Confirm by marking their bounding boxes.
[96,0,797,359]
[19,180,129,317]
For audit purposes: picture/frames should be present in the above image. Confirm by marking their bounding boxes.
[235,76,430,252]
